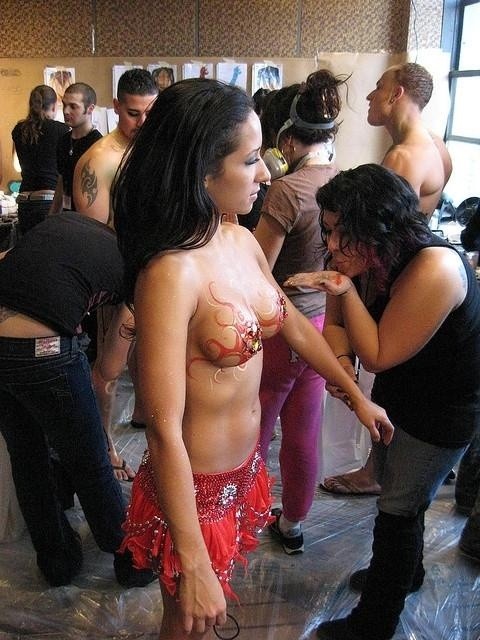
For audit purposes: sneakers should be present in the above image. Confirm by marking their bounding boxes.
[114,554,159,590]
[268,507,305,555]
[455,489,480,562]
[37,530,82,587]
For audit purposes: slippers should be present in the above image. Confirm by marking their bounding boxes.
[130,420,147,428]
[319,474,381,496]
[112,459,137,482]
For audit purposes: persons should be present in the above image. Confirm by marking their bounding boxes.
[258,64,480,640]
[109,79,396,640]
[0,69,158,587]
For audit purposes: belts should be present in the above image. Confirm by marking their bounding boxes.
[16,194,55,204]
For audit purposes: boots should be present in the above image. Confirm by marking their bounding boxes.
[317,500,430,640]
